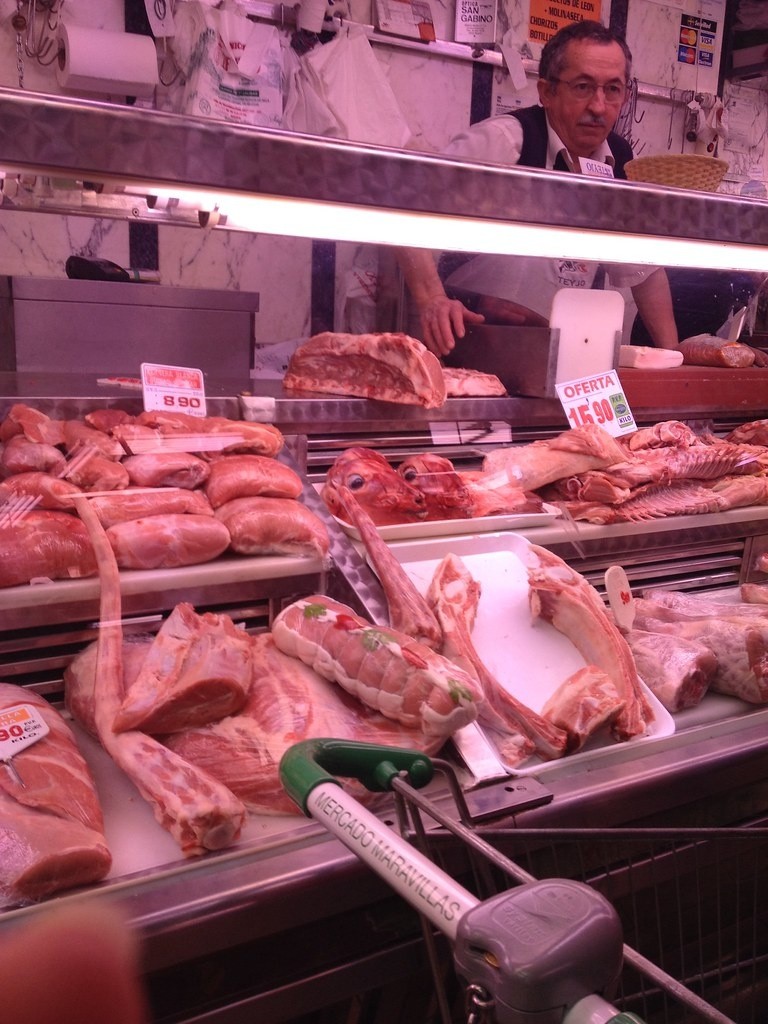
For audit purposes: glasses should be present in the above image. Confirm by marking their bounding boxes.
[550,76,629,105]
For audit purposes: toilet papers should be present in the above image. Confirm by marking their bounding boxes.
[54,23,159,99]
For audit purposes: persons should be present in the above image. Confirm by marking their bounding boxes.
[392,19,679,357]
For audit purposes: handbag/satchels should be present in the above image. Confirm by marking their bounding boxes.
[182,7,410,148]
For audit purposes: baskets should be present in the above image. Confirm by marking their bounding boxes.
[624,155,729,194]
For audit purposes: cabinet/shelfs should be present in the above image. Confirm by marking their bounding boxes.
[1,85,767,974]
